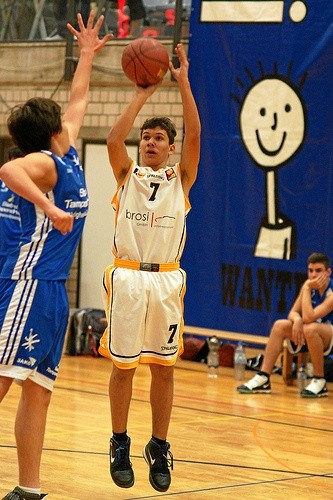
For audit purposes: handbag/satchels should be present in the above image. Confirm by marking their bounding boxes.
[69,307,107,356]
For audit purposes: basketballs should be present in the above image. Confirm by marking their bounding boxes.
[121,37,170,87]
[179,336,205,361]
[217,343,236,368]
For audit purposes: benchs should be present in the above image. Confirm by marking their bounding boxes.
[100,318,308,385]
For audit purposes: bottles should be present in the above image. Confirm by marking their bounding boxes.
[207,335,220,379]
[297,365,306,398]
[233,341,248,382]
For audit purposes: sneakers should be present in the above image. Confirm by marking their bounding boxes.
[109,433,134,488]
[142,438,173,492]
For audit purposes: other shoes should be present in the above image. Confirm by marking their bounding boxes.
[237,371,271,395]
[300,378,329,398]
[0,487,49,500]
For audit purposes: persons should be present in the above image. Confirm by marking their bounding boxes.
[0,7,113,500]
[236,252,333,398]
[96,42,202,493]
[7,146,26,162]
